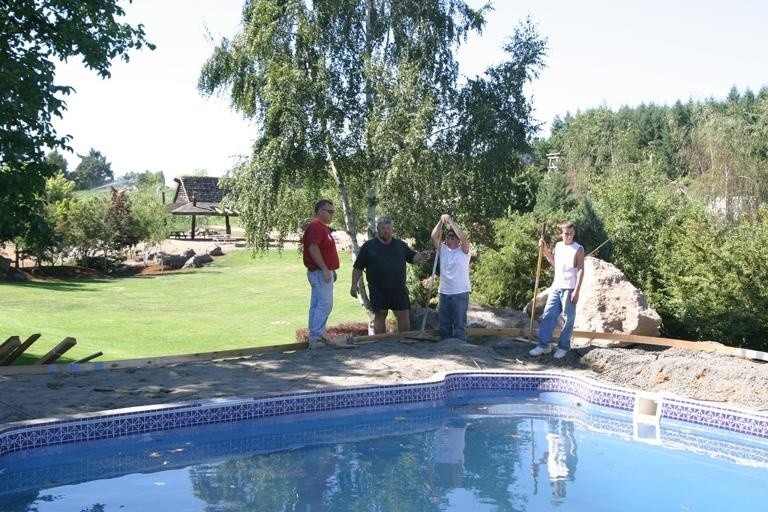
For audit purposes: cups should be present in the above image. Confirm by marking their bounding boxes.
[345,334,354,345]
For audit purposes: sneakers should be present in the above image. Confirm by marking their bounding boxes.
[309,337,336,349]
[554,348,569,358]
[529,344,552,356]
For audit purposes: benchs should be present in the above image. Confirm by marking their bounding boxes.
[167,229,236,245]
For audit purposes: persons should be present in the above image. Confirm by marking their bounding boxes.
[530,416,579,507]
[529,222,585,359]
[417,419,472,504]
[302,200,339,348]
[430,214,471,343]
[350,216,432,335]
[355,431,410,474]
[289,446,336,511]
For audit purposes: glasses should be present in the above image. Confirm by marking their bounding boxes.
[323,209,334,213]
